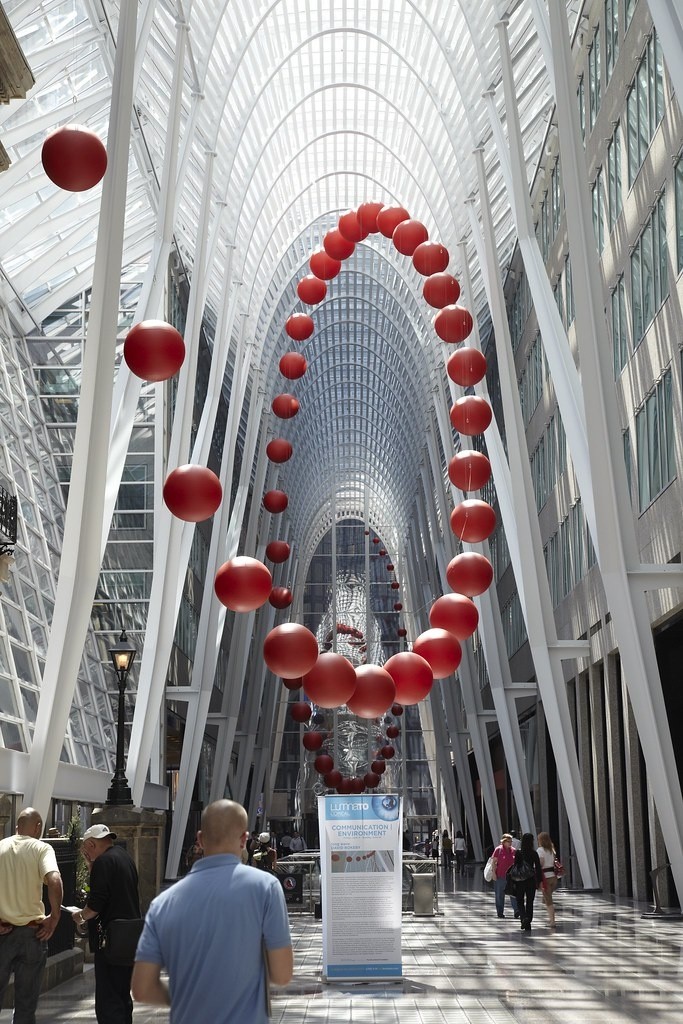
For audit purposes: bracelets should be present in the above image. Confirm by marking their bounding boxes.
[79,911,86,922]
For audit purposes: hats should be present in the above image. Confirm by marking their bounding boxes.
[83,824,117,842]
[259,832,270,843]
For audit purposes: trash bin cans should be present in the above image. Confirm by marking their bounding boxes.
[412,872,435,916]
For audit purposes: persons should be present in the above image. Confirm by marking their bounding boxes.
[454,831,466,875]
[131,800,294,1024]
[424,838,439,860]
[442,834,452,869]
[403,830,410,852]
[246,831,307,874]
[512,833,542,931]
[491,833,519,918]
[533,832,558,927]
[0,808,64,1024]
[72,825,145,1024]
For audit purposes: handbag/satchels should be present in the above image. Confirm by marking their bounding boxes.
[509,850,537,882]
[484,857,497,882]
[98,919,145,967]
[555,860,564,880]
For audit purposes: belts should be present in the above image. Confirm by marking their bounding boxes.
[1,919,39,929]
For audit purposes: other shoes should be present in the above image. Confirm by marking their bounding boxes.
[520,917,532,936]
[546,921,557,928]
[497,914,505,919]
[514,911,520,919]
